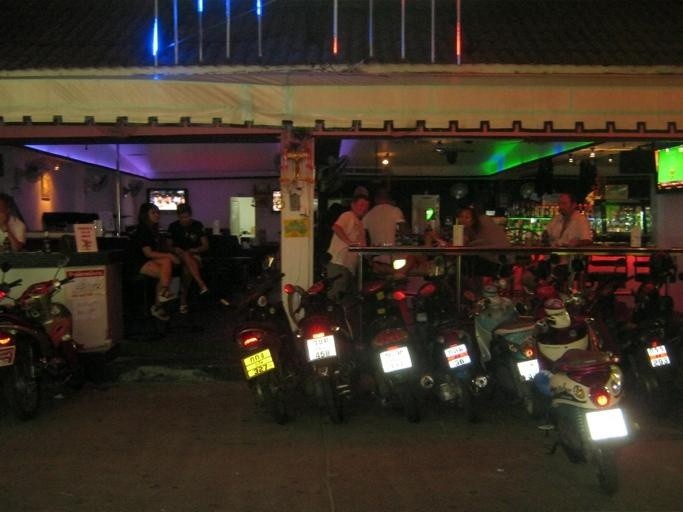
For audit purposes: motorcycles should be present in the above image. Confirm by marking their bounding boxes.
[0,256,80,421]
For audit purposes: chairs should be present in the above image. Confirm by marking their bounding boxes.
[124,239,282,316]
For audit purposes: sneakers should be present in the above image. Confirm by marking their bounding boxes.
[145,282,211,322]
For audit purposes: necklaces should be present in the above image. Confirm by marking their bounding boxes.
[1,226,8,235]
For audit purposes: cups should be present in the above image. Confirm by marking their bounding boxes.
[452,224,464,247]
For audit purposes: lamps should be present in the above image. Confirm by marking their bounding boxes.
[40,172,54,201]
[381,154,389,166]
[568,147,613,166]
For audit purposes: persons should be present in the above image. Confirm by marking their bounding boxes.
[165,205,210,316]
[315,184,593,292]
[0,191,27,252]
[127,203,183,322]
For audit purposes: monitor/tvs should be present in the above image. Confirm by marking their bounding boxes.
[42,211,99,232]
[619,153,654,174]
[652,142,683,194]
[146,187,188,214]
[271,189,281,212]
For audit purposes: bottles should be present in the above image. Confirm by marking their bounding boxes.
[576,203,594,218]
[630,225,642,248]
[503,202,558,241]
